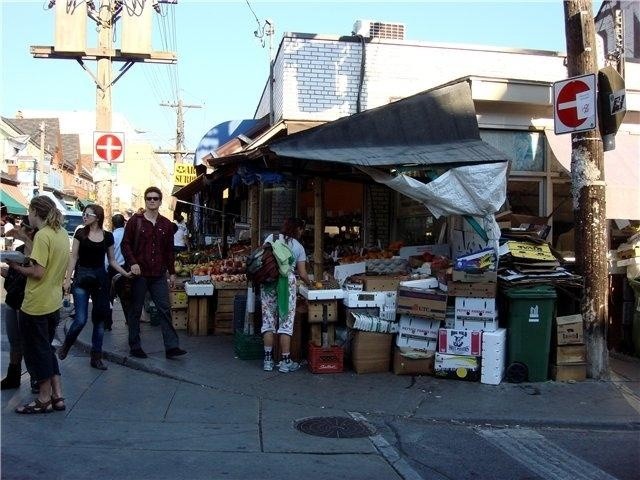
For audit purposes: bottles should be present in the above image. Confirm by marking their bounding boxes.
[63,290,70,312]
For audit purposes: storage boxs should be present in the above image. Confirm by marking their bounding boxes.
[551,314,589,382]
[168,243,505,386]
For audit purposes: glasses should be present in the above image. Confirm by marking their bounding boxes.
[145,197,159,200]
[82,213,96,217]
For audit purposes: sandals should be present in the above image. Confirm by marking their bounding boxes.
[51,395,65,410]
[15,400,53,414]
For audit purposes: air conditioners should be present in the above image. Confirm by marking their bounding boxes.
[352,19,406,42]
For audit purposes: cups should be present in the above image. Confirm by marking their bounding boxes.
[5,234,13,252]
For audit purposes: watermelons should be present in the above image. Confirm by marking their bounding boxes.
[174,264,190,278]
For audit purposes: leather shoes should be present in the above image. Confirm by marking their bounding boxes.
[166,347,188,359]
[129,348,147,358]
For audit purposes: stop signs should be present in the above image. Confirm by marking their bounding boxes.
[552,73,597,135]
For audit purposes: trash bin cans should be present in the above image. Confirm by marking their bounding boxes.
[499,286,557,383]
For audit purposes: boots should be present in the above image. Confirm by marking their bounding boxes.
[59,342,72,360]
[90,351,107,370]
[1,363,21,390]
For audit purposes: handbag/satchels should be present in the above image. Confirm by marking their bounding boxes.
[247,243,279,283]
[115,273,137,299]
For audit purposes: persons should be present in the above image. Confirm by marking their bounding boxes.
[245,218,311,374]
[1,186,192,415]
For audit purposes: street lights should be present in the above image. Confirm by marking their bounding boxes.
[134,126,184,195]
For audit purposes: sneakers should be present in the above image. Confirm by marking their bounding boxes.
[279,360,300,373]
[263,359,275,370]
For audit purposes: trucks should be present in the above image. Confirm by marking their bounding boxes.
[55,212,87,279]
